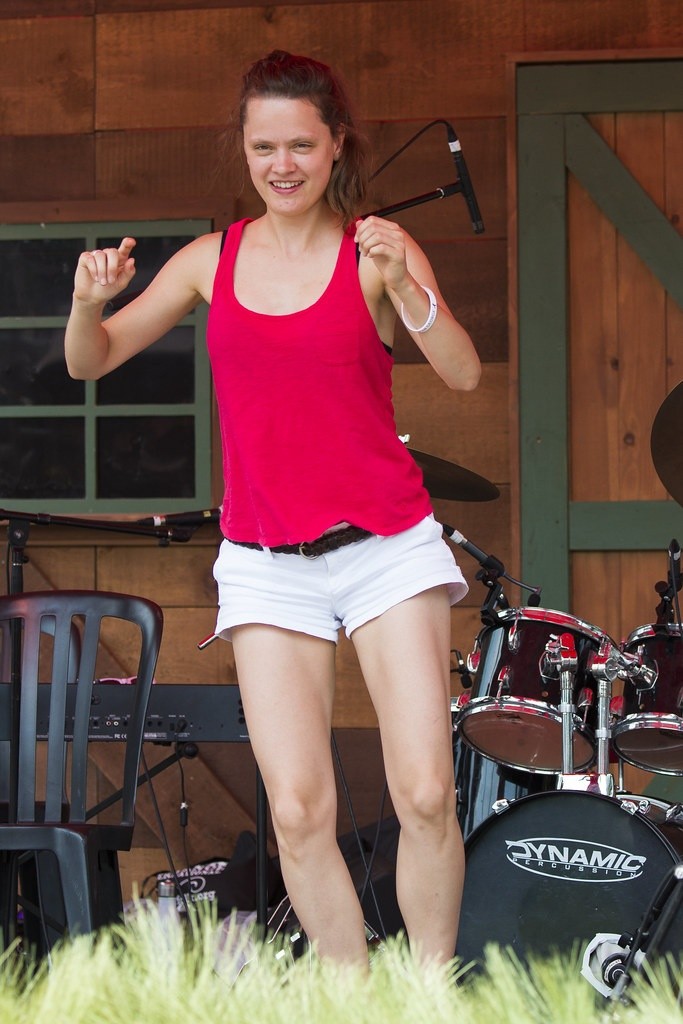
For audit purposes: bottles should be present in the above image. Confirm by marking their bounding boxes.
[158,881,185,953]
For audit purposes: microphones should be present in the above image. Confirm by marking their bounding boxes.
[441,523,505,578]
[447,125,485,235]
[608,963,627,984]
[666,540,683,592]
[138,504,223,527]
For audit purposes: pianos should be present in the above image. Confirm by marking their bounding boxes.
[0,683,251,935]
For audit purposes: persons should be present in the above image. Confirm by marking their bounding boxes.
[62,49,484,977]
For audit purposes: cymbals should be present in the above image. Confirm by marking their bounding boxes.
[406,446,500,502]
[650,381,683,509]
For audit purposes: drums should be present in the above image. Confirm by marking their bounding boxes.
[455,606,617,775]
[450,696,563,842]
[453,789,683,1023]
[611,622,683,778]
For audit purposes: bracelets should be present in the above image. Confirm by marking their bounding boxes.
[399,285,437,333]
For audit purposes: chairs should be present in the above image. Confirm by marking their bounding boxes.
[0,590,163,941]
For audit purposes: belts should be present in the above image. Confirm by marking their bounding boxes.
[225,526,372,560]
